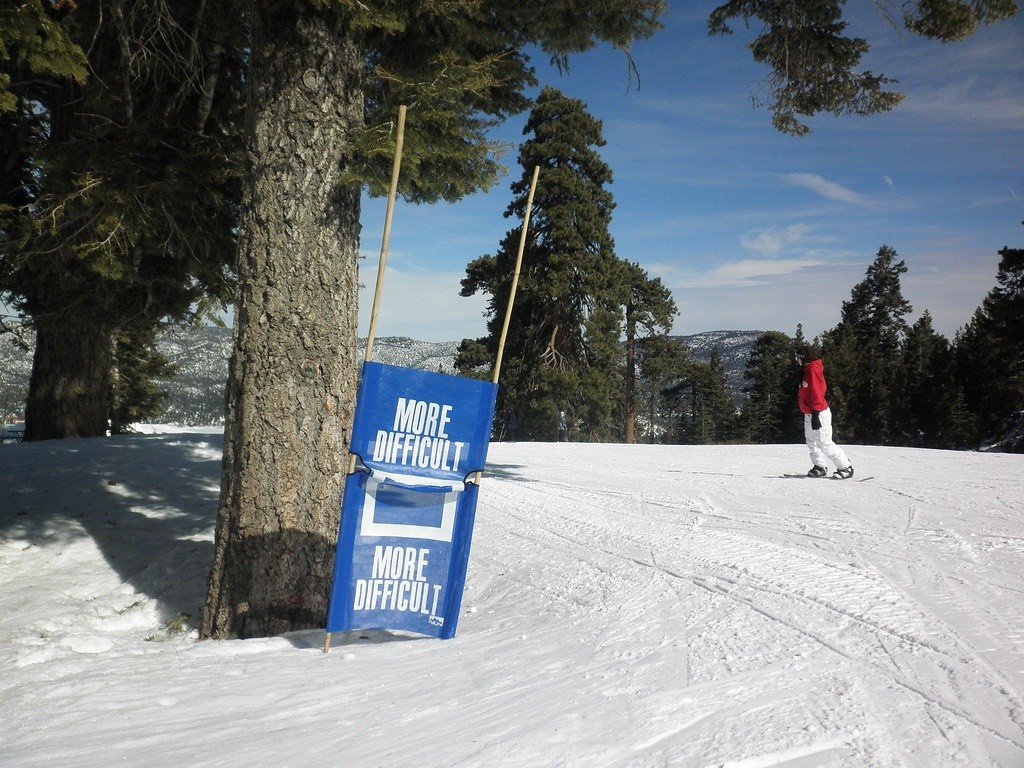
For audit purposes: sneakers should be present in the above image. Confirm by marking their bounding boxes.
[808,464,827,477]
[833,465,853,479]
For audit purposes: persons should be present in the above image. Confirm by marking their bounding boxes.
[795,346,854,479]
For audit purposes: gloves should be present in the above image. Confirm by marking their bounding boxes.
[812,410,822,430]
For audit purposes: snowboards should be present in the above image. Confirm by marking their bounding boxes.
[783,473,875,482]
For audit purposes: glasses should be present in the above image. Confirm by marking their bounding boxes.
[794,353,805,361]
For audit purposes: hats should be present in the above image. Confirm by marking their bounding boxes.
[798,346,818,364]
